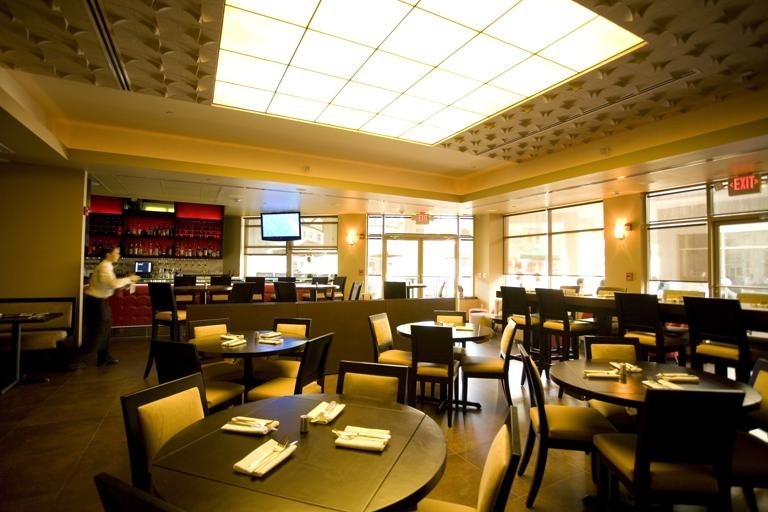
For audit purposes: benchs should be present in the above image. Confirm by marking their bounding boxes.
[0,297,77,375]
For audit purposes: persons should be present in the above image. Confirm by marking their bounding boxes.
[68,244,141,371]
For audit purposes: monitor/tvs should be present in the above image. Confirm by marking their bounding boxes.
[260,211,301,241]
[135,261,152,273]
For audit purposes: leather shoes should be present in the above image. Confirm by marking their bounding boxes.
[98,359,119,366]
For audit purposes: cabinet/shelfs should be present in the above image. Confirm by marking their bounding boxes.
[85,212,224,262]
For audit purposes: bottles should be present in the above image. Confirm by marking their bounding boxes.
[300,414,310,433]
[254,332,259,345]
[620,364,626,375]
[93,223,221,258]
